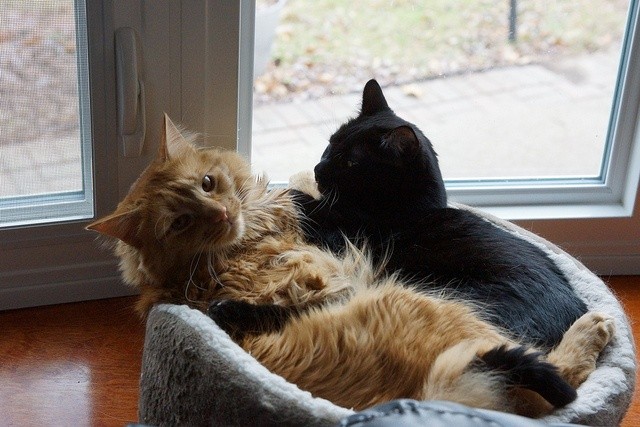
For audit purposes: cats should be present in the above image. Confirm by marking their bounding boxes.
[84,110,617,413]
[267,78,591,409]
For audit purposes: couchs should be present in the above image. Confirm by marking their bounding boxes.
[137,201,637,425]
